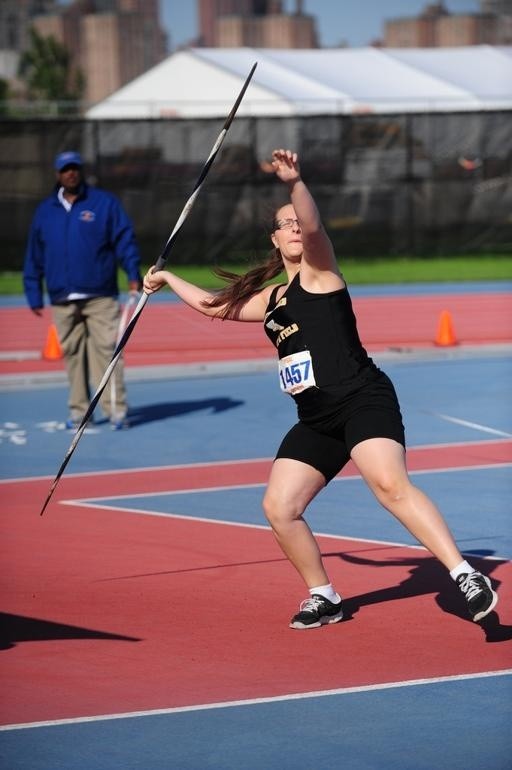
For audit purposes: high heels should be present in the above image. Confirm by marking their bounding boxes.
[42,324,64,361]
[434,310,456,350]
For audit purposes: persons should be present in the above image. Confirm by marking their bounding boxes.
[18,145,145,433]
[135,147,502,632]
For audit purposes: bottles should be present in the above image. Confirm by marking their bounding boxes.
[54,151,81,171]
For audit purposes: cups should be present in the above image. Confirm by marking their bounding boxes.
[274,219,300,230]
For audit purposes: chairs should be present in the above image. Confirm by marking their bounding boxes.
[66,416,94,428]
[111,419,129,430]
[456,569,498,622]
[288,592,343,628]
